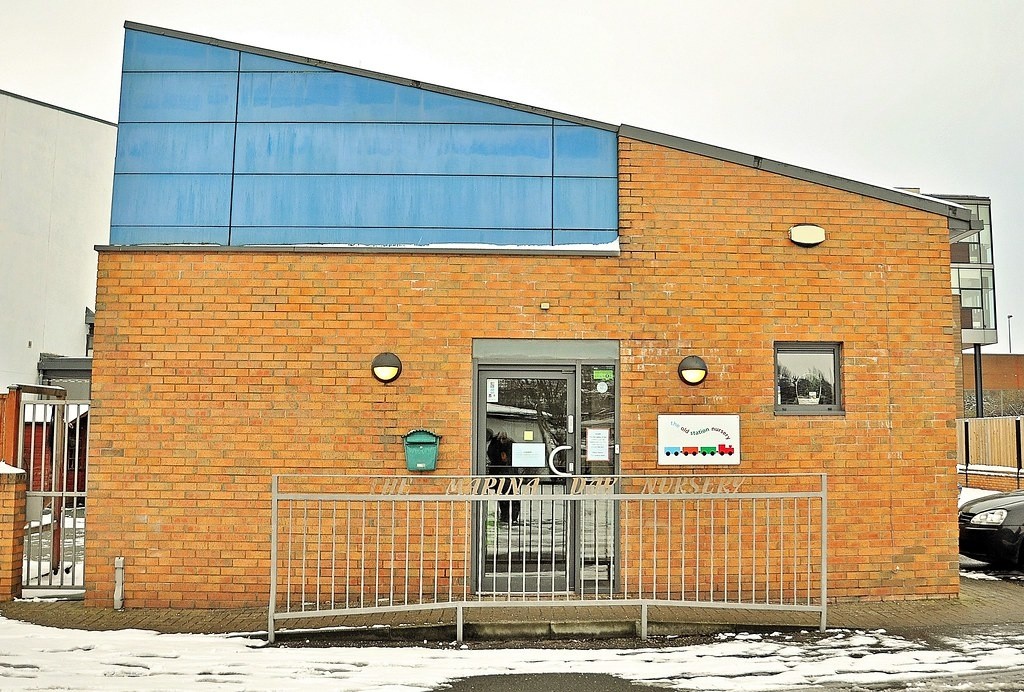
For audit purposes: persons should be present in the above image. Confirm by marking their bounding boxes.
[487,430,523,526]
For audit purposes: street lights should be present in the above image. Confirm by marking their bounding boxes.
[1007,312,1015,355]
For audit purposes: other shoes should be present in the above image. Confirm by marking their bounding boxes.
[501,521,519,526]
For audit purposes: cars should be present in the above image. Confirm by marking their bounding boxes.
[957,489,1024,580]
